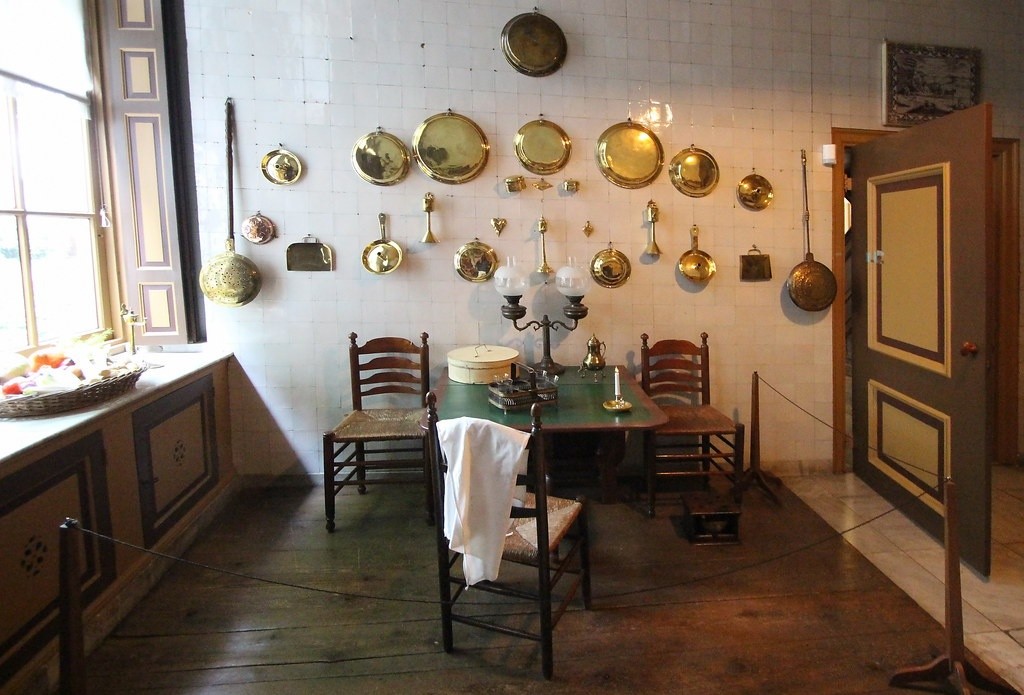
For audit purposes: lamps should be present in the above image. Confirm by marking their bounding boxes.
[494,255,592,375]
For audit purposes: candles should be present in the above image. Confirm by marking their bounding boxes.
[615,366,620,395]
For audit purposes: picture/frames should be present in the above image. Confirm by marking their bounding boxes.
[881,37,981,128]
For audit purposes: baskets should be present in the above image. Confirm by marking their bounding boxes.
[488,362,558,415]
[0,362,150,417]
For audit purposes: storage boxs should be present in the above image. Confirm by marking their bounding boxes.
[681,492,743,547]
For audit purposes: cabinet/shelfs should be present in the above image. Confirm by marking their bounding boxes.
[0,358,237,695]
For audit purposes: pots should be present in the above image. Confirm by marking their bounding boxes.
[361,213,402,275]
[679,224,717,283]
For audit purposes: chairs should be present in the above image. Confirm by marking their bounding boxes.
[640,332,744,519]
[323,332,436,533]
[426,391,592,681]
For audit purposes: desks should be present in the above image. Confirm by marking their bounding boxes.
[417,365,670,560]
